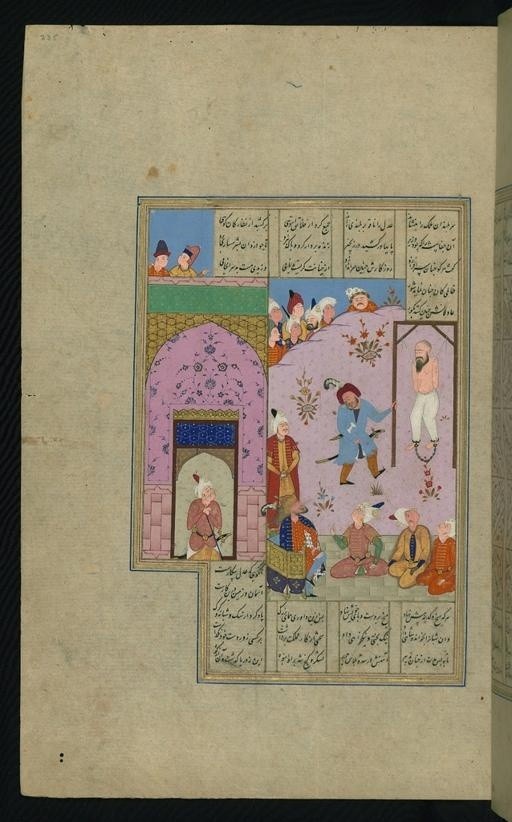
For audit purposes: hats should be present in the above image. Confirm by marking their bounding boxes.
[271,408,289,434]
[361,501,385,526]
[445,517,455,537]
[193,474,213,499]
[323,376,361,405]
[344,288,369,297]
[153,240,170,257]
[183,246,200,267]
[389,508,409,527]
[268,287,337,332]
[274,493,297,514]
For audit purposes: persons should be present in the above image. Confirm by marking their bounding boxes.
[148,240,171,277]
[185,479,223,561]
[152,246,210,278]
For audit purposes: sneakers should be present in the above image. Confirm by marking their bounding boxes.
[340,480,354,485]
[374,469,385,478]
